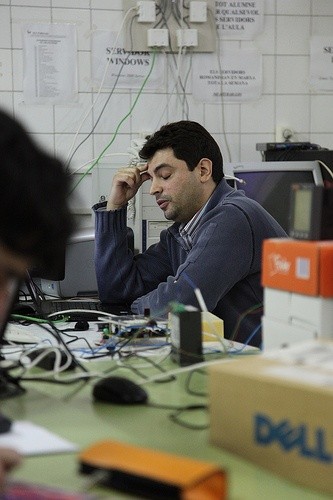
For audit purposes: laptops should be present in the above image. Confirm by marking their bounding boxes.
[22,269,135,321]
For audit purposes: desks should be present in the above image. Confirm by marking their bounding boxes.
[0,295,333,500]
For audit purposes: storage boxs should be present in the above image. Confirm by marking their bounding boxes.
[208,238,333,491]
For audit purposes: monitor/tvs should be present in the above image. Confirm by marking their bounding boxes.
[232,162,324,235]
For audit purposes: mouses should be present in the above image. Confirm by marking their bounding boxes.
[93,376,147,405]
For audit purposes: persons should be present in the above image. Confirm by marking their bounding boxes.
[0,108,78,497]
[91,121,288,350]
